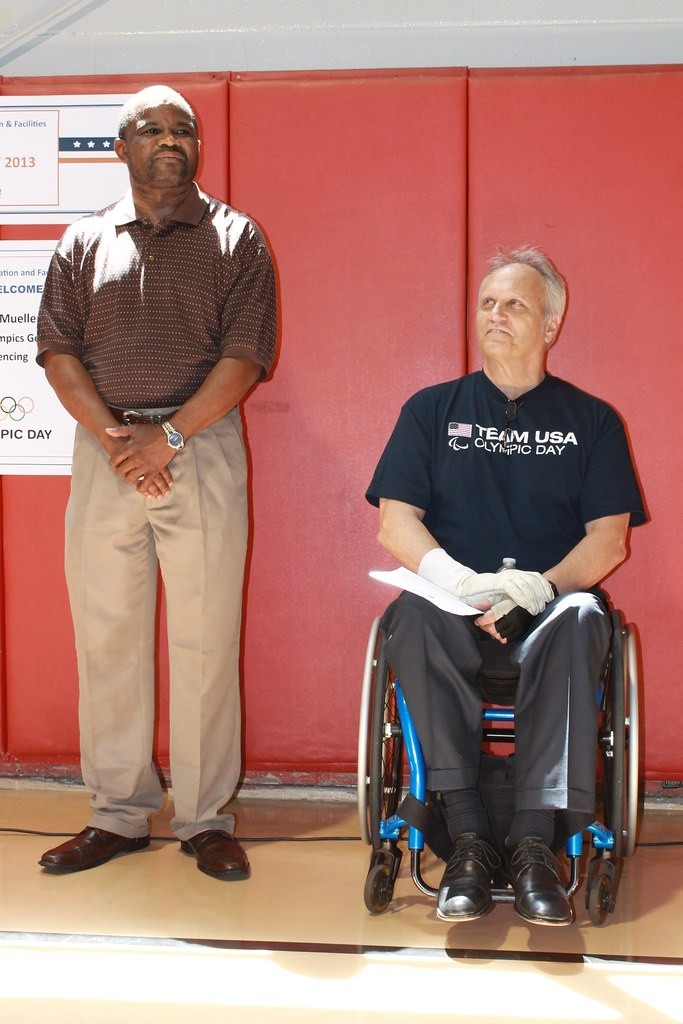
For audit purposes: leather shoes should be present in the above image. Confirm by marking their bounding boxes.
[181,830,251,878]
[501,835,572,926]
[436,832,501,921]
[38,825,150,869]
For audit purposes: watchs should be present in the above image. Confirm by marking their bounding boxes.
[162,421,185,451]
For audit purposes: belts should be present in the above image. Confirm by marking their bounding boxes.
[109,407,178,426]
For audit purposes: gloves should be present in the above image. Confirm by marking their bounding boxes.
[415,547,555,616]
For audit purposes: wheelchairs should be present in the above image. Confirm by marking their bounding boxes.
[356,603,649,926]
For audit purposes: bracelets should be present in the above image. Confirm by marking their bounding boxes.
[544,578,560,596]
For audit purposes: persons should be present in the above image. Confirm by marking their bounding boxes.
[365,245,648,926]
[36,84,276,882]
